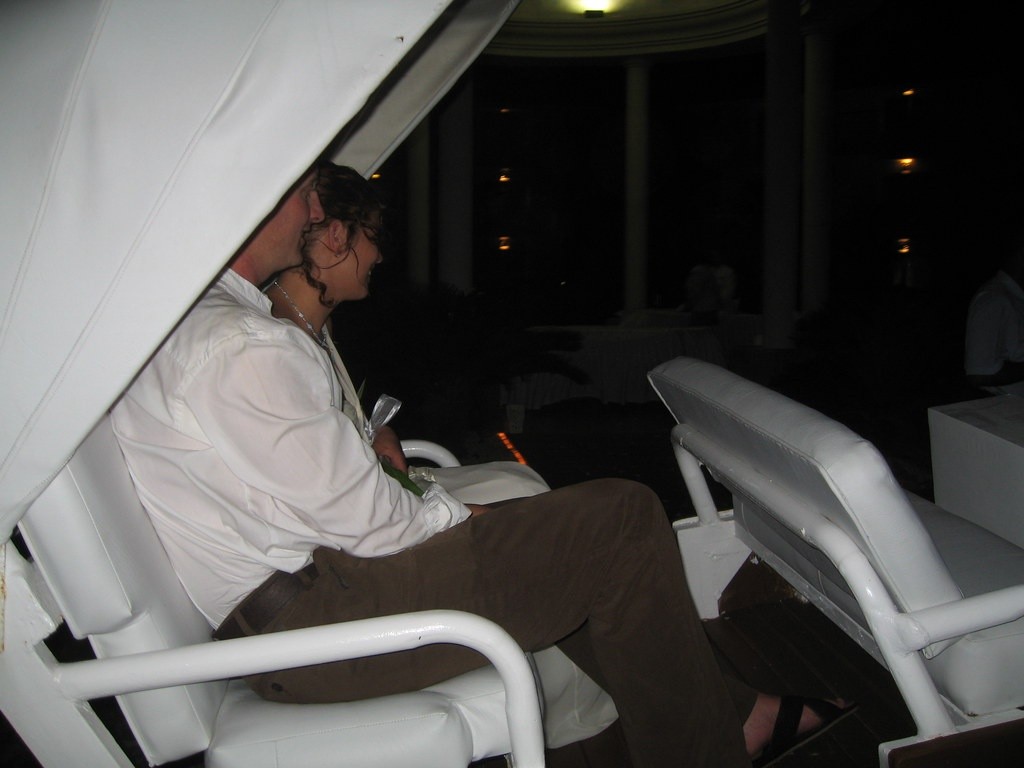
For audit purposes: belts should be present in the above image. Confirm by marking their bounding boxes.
[215,560,318,642]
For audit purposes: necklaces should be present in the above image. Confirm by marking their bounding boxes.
[274,281,327,347]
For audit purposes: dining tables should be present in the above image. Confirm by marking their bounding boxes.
[496,323,728,411]
[718,309,802,350]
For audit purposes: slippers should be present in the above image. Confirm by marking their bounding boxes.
[748,696,858,768]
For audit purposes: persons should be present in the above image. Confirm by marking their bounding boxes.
[110,159,859,768]
[963,231,1024,386]
[261,167,551,505]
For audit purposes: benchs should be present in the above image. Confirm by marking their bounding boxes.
[646,356,1024,768]
[0,413,620,768]
